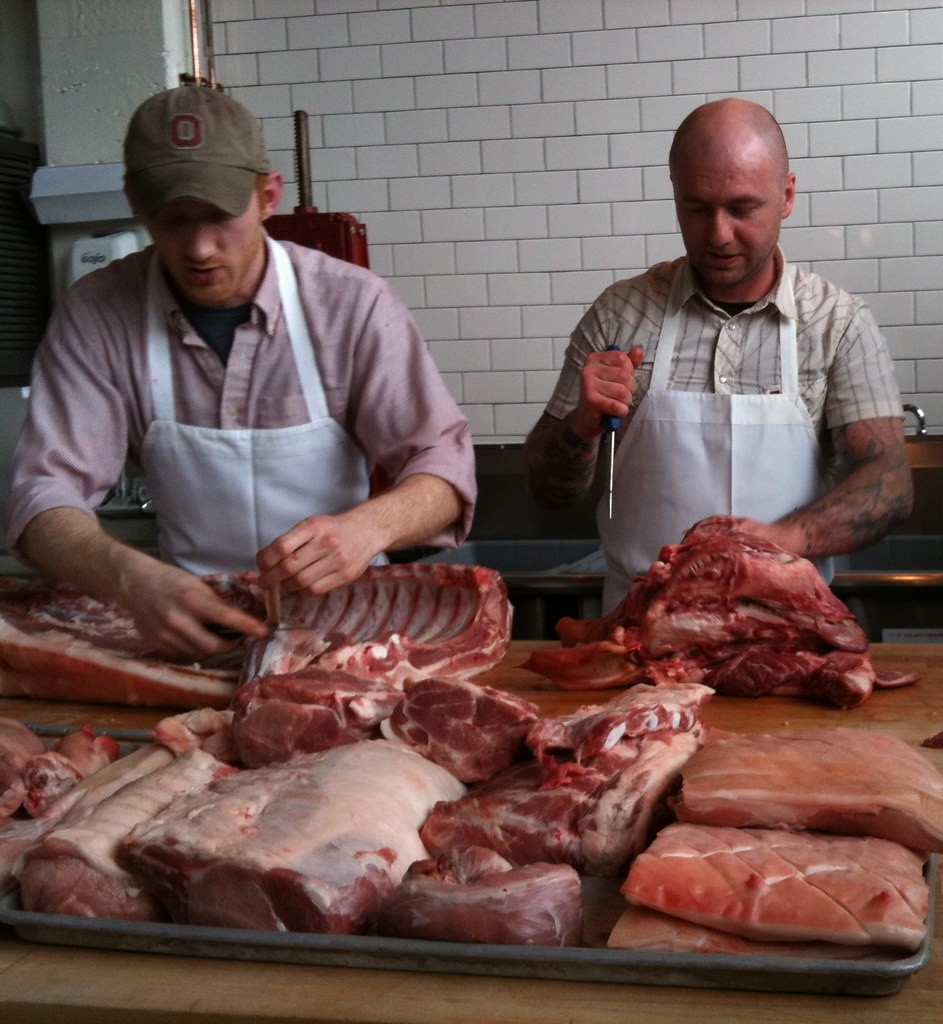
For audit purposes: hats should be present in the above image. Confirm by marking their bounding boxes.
[122,85,270,216]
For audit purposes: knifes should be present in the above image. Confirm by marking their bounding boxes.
[601,345,622,519]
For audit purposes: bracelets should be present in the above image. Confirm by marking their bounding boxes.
[561,414,597,454]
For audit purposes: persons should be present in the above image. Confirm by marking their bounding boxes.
[524,99,915,622]
[1,85,477,659]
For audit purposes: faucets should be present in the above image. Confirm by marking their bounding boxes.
[902,402,926,434]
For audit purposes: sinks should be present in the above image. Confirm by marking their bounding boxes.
[832,533,942,571]
[425,540,602,577]
